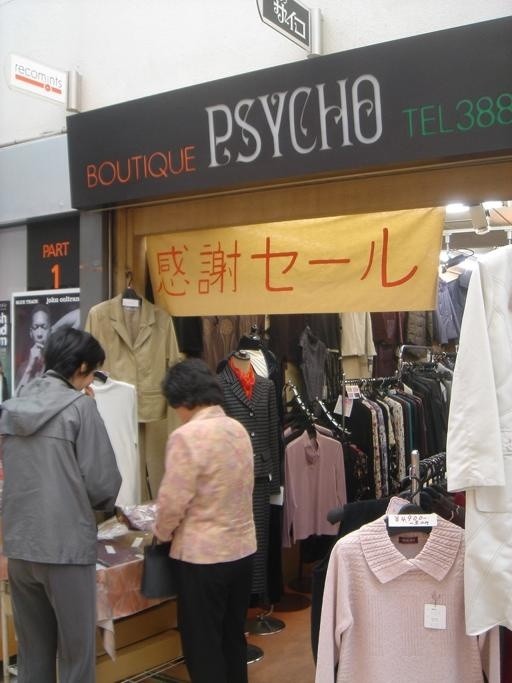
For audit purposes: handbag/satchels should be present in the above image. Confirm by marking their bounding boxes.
[139,534,182,602]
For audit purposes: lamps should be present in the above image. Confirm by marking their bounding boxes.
[469,203,491,235]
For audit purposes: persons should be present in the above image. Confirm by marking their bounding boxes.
[1,326,124,682]
[144,356,260,681]
[14,303,81,397]
[207,350,282,602]
[215,332,284,433]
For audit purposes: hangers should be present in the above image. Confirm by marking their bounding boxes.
[283,351,454,536]
